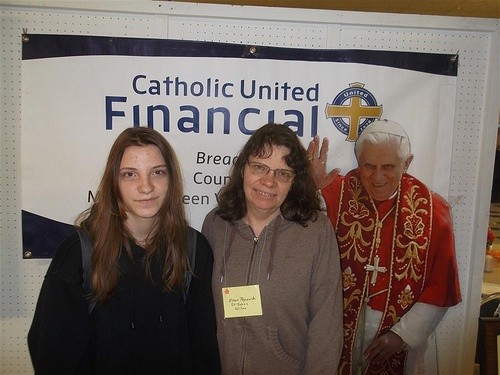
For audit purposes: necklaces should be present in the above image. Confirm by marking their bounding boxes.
[364,203,395,287]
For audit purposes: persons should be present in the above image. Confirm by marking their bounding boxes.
[27,127,221,375]
[202,123,343,375]
[306,119,463,375]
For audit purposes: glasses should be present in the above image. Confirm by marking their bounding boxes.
[247,159,298,183]
